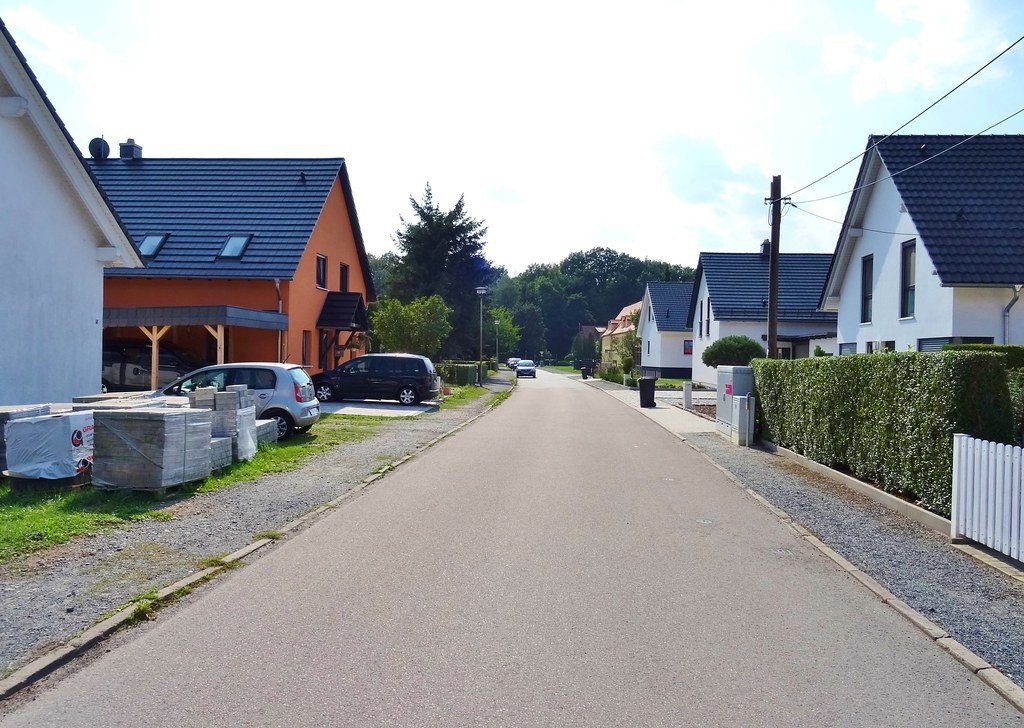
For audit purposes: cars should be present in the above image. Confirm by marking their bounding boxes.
[515,360,538,378]
[153,360,322,442]
[309,352,441,407]
[506,358,522,371]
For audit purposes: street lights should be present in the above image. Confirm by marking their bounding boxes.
[473,286,489,388]
[492,318,501,371]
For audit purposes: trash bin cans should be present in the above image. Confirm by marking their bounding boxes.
[637,377,658,408]
[580,367,588,379]
